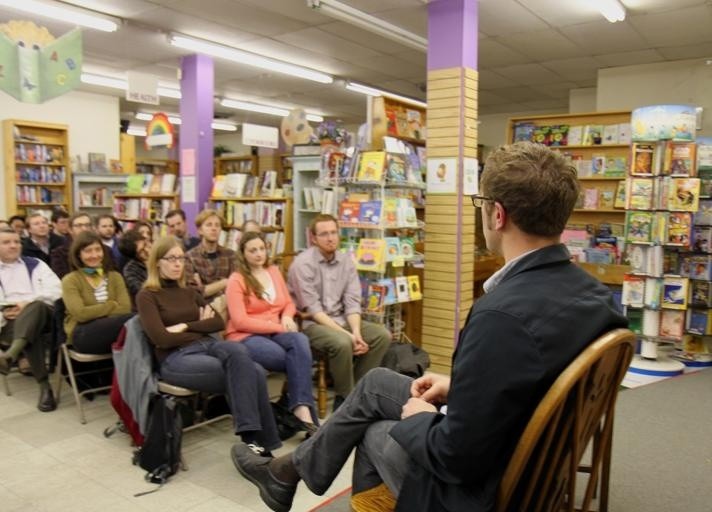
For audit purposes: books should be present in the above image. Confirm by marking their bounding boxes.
[620,138,710,340]
[514,122,630,146]
[13,126,64,211]
[320,94,427,328]
[565,154,626,211]
[83,174,178,231]
[0,27,83,105]
[560,222,626,264]
[215,160,288,260]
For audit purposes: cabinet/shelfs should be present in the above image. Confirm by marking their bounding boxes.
[112,194,182,228]
[204,195,302,278]
[0,119,73,221]
[504,113,630,312]
[215,156,258,178]
[135,159,180,176]
[282,156,321,252]
[73,174,130,218]
[321,96,495,339]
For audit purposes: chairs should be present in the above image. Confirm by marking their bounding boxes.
[351,327,640,512]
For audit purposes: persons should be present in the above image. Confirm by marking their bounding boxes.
[0,204,238,418]
[286,212,392,415]
[227,236,323,437]
[136,232,284,459]
[229,139,627,510]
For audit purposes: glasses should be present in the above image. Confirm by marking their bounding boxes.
[71,223,94,227]
[471,193,494,209]
[160,255,186,263]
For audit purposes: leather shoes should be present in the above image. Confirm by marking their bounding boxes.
[37,380,58,413]
[275,408,321,436]
[228,442,298,511]
[0,353,13,376]
